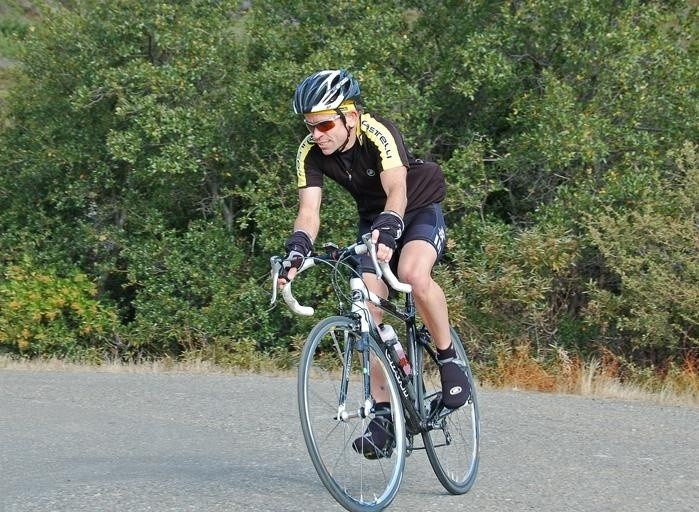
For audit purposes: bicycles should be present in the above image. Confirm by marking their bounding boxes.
[269,233,481,512]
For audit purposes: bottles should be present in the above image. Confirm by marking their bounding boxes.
[378,322,414,382]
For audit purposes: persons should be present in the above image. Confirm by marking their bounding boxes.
[267,69,471,454]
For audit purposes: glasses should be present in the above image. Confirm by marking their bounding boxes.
[303,112,345,134]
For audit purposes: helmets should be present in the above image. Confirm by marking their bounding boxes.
[293,69,360,115]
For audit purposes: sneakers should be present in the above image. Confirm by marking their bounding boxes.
[435,340,472,410]
[352,402,392,453]
